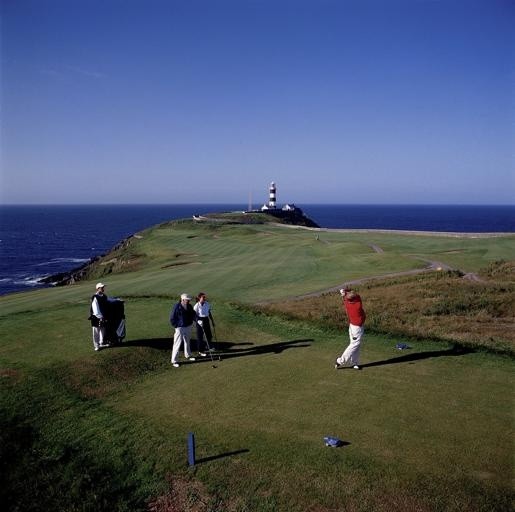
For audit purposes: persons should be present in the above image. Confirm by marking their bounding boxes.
[170,293,216,367]
[88,282,110,351]
[334,289,366,369]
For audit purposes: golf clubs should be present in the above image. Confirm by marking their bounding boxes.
[213,326,221,361]
[342,297,358,340]
[202,326,218,368]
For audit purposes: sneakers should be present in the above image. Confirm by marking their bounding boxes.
[172,347,215,368]
[94,344,109,351]
[334,362,359,370]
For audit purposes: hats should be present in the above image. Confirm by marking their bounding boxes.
[181,293,192,301]
[95,283,108,289]
